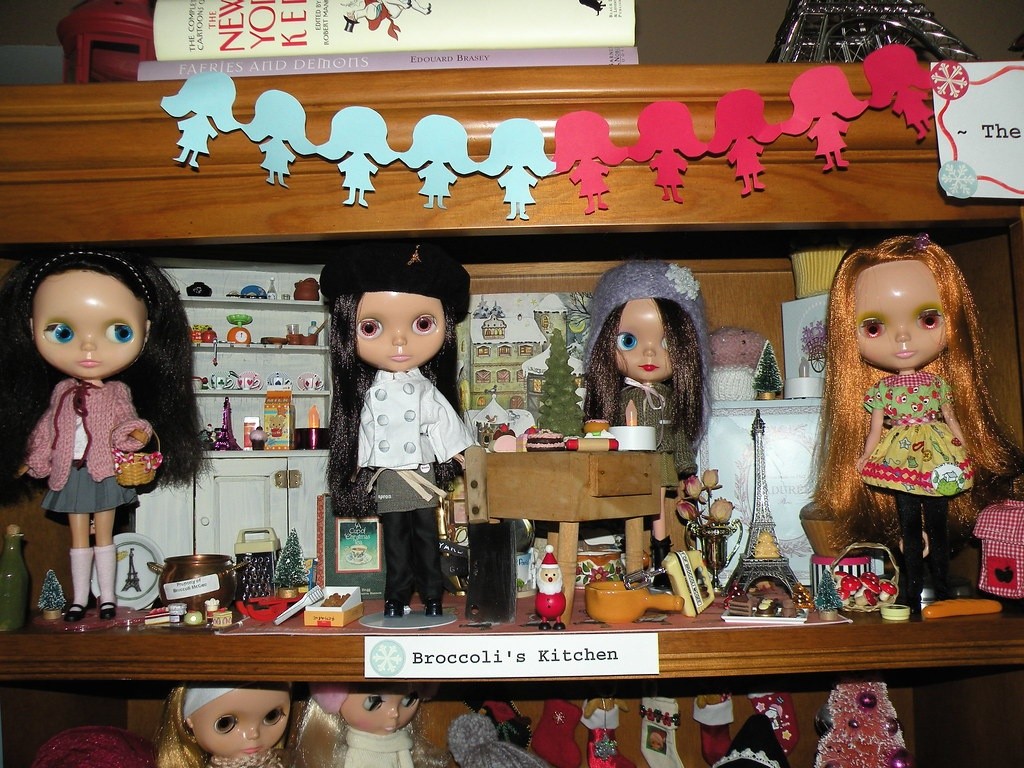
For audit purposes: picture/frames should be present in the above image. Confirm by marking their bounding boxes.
[334,516,382,575]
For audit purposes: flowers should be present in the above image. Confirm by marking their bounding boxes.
[675,466,736,525]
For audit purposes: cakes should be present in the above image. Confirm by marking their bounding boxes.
[526,431,565,453]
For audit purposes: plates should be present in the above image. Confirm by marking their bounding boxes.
[91,533,165,611]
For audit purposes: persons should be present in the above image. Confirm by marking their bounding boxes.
[296,682,434,768]
[1,240,206,620]
[582,260,716,591]
[155,679,291,768]
[812,232,1011,614]
[316,244,477,621]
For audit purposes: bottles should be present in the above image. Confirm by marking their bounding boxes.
[0,524,31,632]
[266,277,277,300]
[308,321,318,346]
[309,405,320,428]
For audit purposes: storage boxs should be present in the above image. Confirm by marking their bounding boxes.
[304,586,364,628]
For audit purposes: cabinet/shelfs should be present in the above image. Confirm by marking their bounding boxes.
[135,256,335,558]
[0,59,1024,768]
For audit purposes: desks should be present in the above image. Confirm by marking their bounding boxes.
[463,452,663,624]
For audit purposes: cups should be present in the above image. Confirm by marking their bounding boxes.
[304,378,324,391]
[193,379,203,389]
[211,377,233,389]
[238,378,260,390]
[287,324,299,335]
[273,377,291,386]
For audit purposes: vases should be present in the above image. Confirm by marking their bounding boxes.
[684,519,743,593]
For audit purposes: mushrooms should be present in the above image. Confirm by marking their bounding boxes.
[833,571,897,607]
